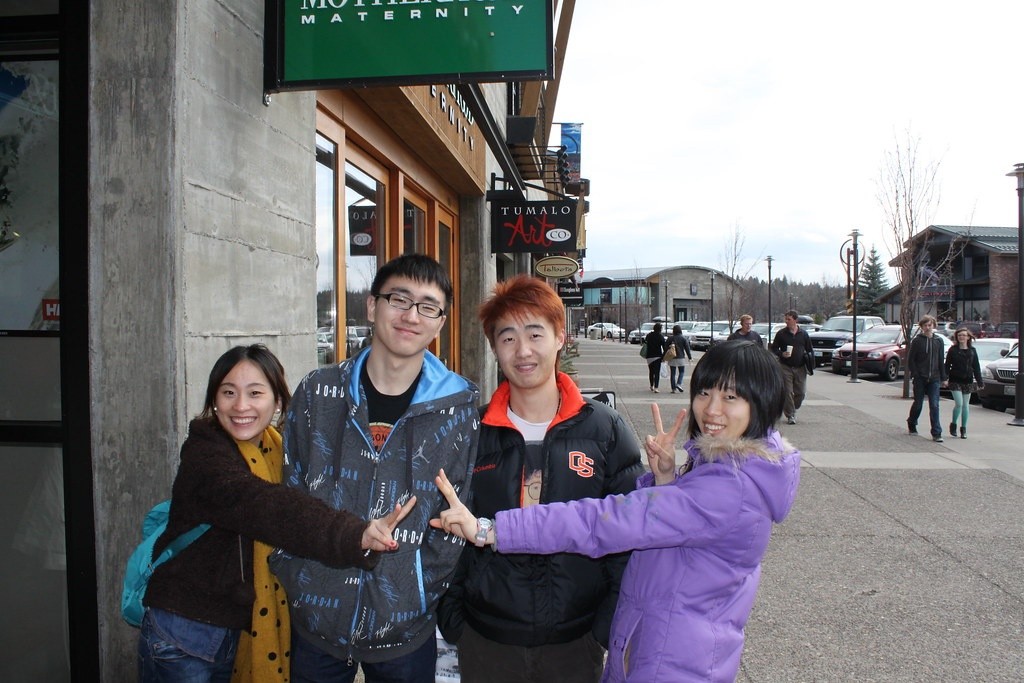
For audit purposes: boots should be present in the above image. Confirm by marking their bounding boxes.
[960,427,967,439]
[950,423,957,436]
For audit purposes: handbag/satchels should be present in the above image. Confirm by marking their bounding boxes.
[663,336,676,361]
[640,341,648,358]
[659,361,669,378]
[120,498,211,629]
[804,352,814,375]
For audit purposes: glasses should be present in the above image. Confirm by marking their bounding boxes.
[375,292,444,319]
[957,335,968,337]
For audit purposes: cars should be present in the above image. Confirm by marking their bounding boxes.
[583,322,625,338]
[940,337,1019,392]
[933,320,1020,356]
[317,317,372,364]
[832,323,923,381]
[976,343,1018,412]
[628,316,823,351]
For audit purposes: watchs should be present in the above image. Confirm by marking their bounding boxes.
[474,517,492,547]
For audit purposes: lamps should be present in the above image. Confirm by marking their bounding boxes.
[505,142,572,188]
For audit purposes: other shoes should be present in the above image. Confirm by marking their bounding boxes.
[671,390,675,393]
[649,386,654,391]
[788,416,796,424]
[933,435,943,442]
[908,424,918,436]
[676,384,683,392]
[655,388,660,392]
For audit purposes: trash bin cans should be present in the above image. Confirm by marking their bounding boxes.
[590,328,598,340]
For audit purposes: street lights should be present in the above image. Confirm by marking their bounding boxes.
[846,228,864,383]
[1005,162,1024,428]
[793,296,799,310]
[787,291,794,310]
[600,293,606,340]
[617,291,624,343]
[763,255,776,352]
[623,286,630,344]
[662,280,671,336]
[707,270,717,348]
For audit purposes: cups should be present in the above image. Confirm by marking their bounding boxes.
[786,345,794,357]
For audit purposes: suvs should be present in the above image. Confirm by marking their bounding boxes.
[807,315,887,363]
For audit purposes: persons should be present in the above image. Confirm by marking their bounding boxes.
[137,345,417,683]
[726,314,763,349]
[644,323,665,393]
[428,338,800,683]
[666,325,693,393]
[772,310,814,425]
[267,254,482,683]
[433,273,647,683]
[944,328,985,439]
[907,315,945,442]
